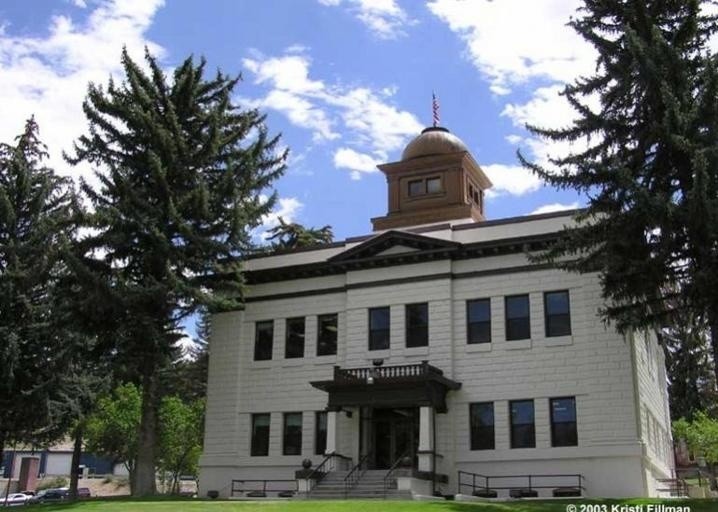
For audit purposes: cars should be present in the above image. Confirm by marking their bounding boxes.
[0,487,91,507]
